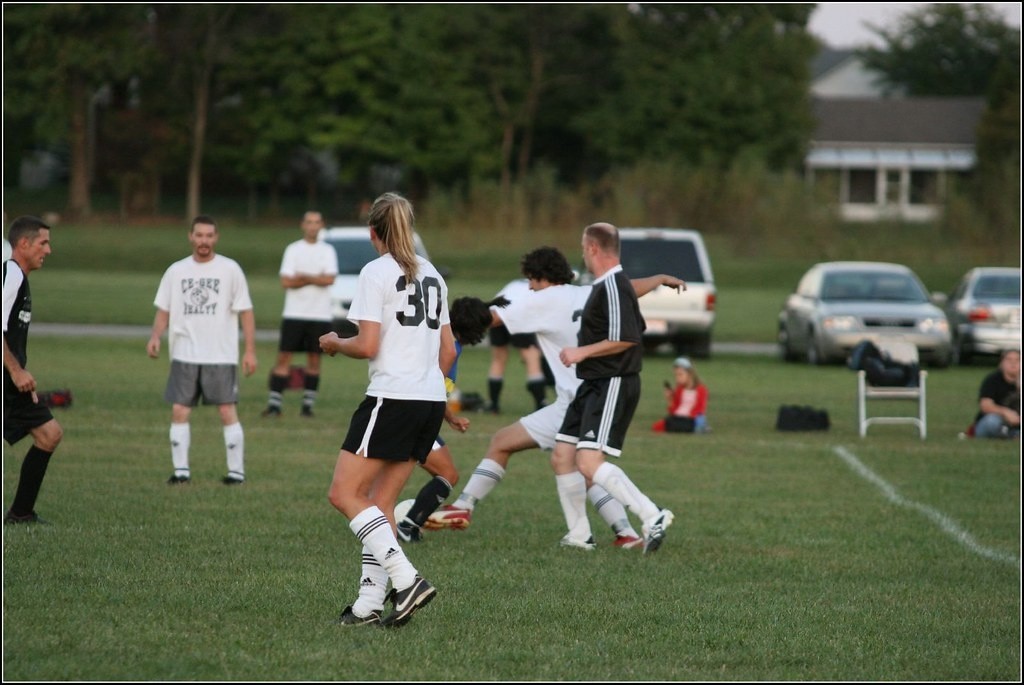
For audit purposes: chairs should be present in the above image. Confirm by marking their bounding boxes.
[858,341,927,440]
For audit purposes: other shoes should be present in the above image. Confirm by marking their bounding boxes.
[167,474,188,486]
[222,475,240,485]
[4,512,51,525]
[300,407,314,420]
[262,405,279,417]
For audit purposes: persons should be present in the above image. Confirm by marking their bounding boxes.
[319,192,457,632]
[421,246,687,549]
[146,217,258,488]
[652,354,709,433]
[551,223,675,555]
[395,339,470,545]
[958,348,1021,440]
[258,209,339,418]
[2,217,64,523]
[483,278,547,415]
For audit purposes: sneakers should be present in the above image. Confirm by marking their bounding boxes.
[335,605,382,628]
[558,535,596,551]
[397,520,422,543]
[379,574,438,629]
[610,536,644,549]
[642,508,675,557]
[423,504,471,531]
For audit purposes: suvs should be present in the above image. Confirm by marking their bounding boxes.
[570,226,718,359]
[323,226,430,338]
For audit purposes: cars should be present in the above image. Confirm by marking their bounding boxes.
[777,261,952,369]
[942,266,1020,366]
